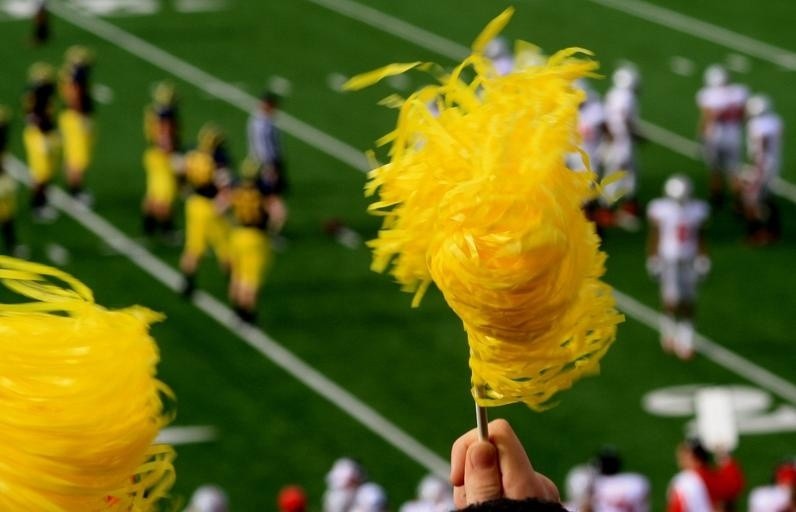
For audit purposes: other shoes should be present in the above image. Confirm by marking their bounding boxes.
[232,304,257,322]
[74,194,95,205]
[28,194,58,218]
[180,273,198,301]
[143,212,176,236]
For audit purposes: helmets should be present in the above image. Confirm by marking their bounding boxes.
[609,63,771,200]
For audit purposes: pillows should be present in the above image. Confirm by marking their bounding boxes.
[609,63,771,200]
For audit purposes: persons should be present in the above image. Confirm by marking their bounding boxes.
[321,458,387,509]
[448,419,565,509]
[245,92,287,235]
[644,177,713,360]
[400,472,455,509]
[663,439,746,510]
[58,45,96,209]
[32,3,52,47]
[563,450,651,508]
[278,485,307,509]
[227,156,289,324]
[693,64,791,247]
[140,81,187,238]
[1,105,24,257]
[21,61,63,225]
[418,36,653,236]
[180,123,236,301]
[748,460,793,509]
[188,483,230,508]
